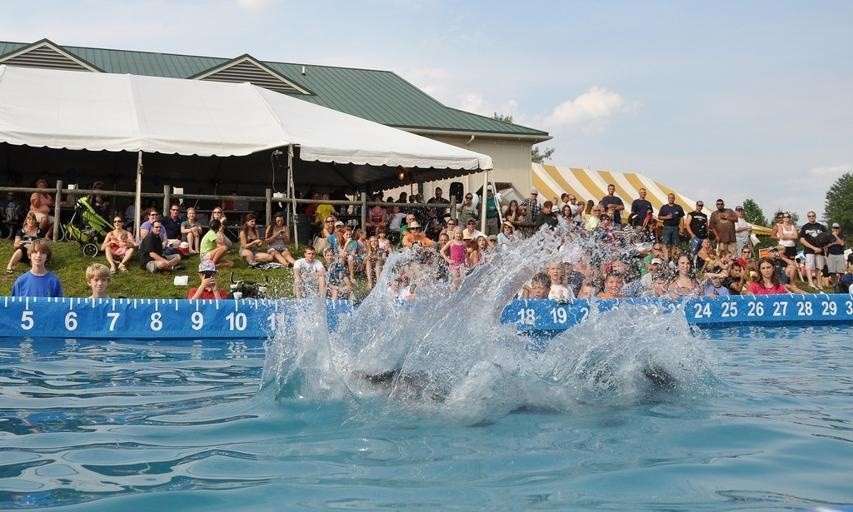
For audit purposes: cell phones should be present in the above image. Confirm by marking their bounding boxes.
[204,270,211,278]
[279,230,284,233]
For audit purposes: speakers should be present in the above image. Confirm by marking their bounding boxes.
[449,182,463,204]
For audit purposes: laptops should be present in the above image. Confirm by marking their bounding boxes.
[232,200,248,211]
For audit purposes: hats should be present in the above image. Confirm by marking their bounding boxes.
[198,260,215,273]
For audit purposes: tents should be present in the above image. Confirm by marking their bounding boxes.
[534,163,773,236]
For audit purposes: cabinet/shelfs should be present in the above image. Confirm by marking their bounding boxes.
[169,208,261,242]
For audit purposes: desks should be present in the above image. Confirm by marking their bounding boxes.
[50,203,103,240]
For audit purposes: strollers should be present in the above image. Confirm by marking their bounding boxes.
[62,193,115,258]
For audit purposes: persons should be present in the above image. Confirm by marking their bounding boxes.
[354,366,688,429]
[476,185,853,299]
[1,180,501,298]
